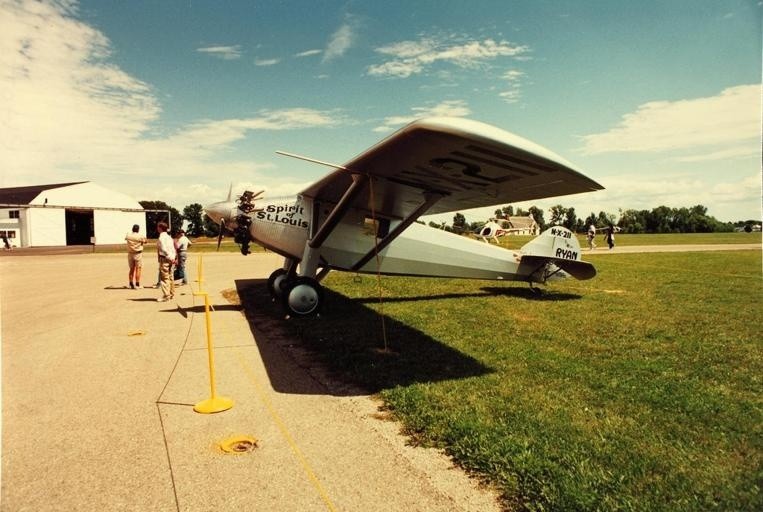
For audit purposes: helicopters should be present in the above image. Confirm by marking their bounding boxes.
[473,217,536,244]
[596,225,622,233]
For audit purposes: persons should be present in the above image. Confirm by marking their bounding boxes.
[603,223,616,250]
[175,230,191,286]
[153,221,176,303]
[586,221,597,252]
[153,228,180,289]
[123,223,148,290]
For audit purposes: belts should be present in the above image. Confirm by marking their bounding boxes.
[179,250,187,253]
[128,251,143,254]
[159,254,166,259]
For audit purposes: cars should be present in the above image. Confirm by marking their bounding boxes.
[735,225,761,232]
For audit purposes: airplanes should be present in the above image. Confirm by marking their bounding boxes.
[205,117,606,318]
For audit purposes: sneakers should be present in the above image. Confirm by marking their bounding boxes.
[128,282,143,289]
[152,280,162,289]
[157,295,173,302]
